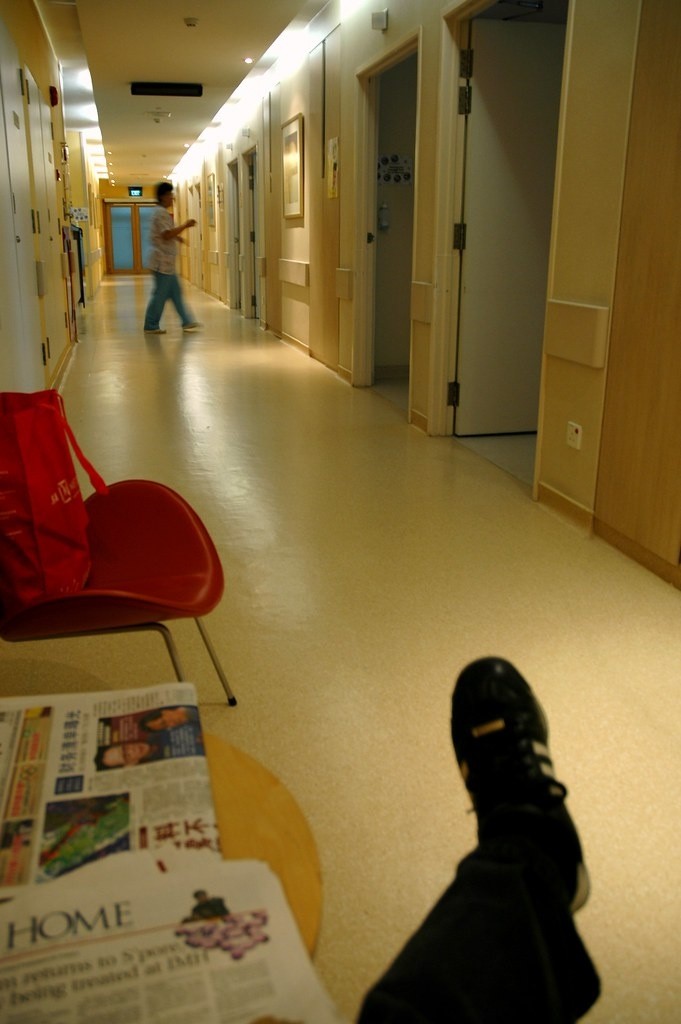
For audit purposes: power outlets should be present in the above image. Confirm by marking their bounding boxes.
[566,421,583,451]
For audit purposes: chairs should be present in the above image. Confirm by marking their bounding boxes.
[0,479,239,707]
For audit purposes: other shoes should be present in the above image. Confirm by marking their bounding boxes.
[183,322,203,332]
[143,328,165,333]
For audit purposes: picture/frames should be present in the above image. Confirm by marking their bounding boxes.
[279,112,304,219]
[205,172,215,226]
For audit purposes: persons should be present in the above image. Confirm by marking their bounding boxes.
[144,183,202,335]
[95,705,226,921]
[358,657,602,1024]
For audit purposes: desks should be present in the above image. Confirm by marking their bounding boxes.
[200,728,324,963]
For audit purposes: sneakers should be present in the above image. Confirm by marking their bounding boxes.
[450,656,590,915]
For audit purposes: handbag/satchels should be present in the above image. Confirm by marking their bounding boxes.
[0,390,111,603]
